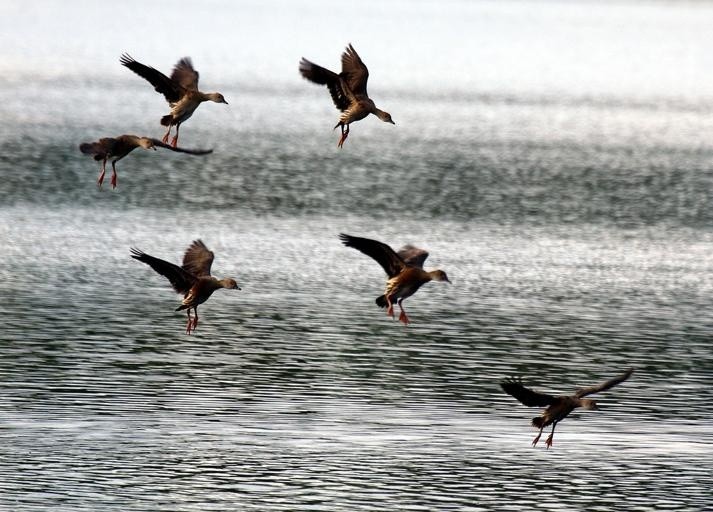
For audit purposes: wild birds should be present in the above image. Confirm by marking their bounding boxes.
[297,42,396,149]
[128,239,242,335]
[118,51,229,148]
[338,232,452,326]
[498,367,634,451]
[79,134,214,191]
